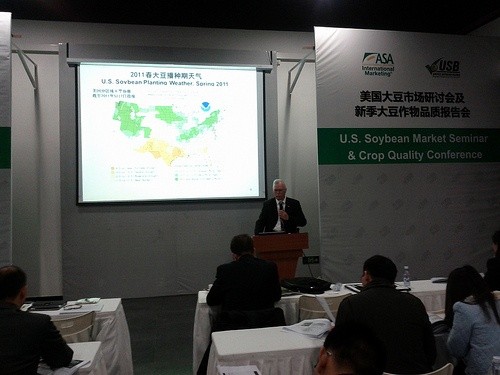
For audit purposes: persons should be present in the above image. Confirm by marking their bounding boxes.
[0,265,74,375]
[206,234,287,332]
[486,234,500,291]
[321,255,436,375]
[254,179,307,235]
[444,265,500,375]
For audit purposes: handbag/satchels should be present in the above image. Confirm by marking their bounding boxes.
[281,277,333,295]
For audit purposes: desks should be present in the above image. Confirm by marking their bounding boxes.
[20,298,134,375]
[206,310,444,375]
[192,273,485,375]
[36,340,109,375]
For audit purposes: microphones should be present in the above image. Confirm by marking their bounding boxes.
[278,200,283,219]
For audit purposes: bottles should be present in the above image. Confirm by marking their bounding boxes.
[404,266,411,287]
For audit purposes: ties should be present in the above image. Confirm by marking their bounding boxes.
[278,202,285,231]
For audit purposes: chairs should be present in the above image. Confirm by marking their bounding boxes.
[297,292,351,323]
[208,306,287,333]
[50,310,96,343]
